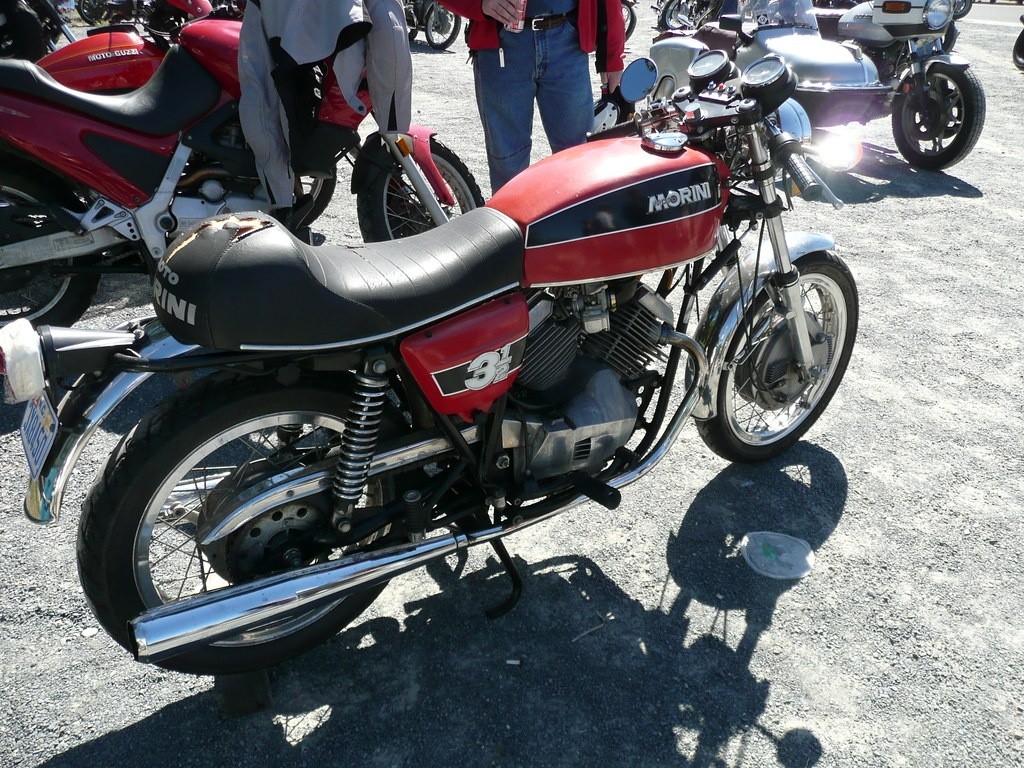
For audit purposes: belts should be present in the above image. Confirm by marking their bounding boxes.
[525,7,578,30]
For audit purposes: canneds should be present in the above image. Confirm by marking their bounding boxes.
[504,0,527,34]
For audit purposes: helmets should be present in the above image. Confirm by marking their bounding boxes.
[590,100,621,136]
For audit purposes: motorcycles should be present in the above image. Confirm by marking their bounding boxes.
[0,0,492,339]
[649,1,989,171]
[14,0,861,687]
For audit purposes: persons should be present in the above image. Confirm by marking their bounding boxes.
[438,0,626,196]
